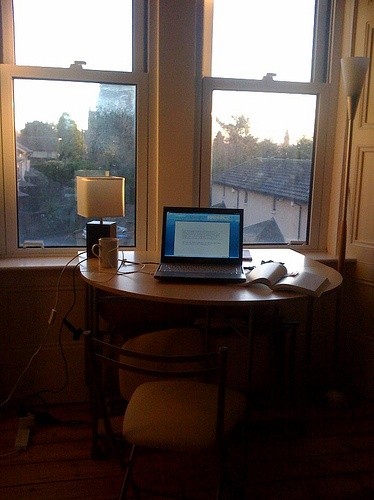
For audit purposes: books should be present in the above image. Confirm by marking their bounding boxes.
[239,262,331,298]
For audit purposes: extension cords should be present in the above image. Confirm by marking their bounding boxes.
[14,428,30,450]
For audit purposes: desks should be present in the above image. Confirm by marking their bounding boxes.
[78,248,344,461]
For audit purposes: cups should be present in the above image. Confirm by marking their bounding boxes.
[92,237,119,268]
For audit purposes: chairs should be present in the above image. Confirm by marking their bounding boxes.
[62,312,305,500]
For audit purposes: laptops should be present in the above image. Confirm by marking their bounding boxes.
[154,206,247,282]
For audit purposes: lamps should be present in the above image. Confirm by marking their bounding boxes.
[76,175,125,258]
[322,55,372,412]
